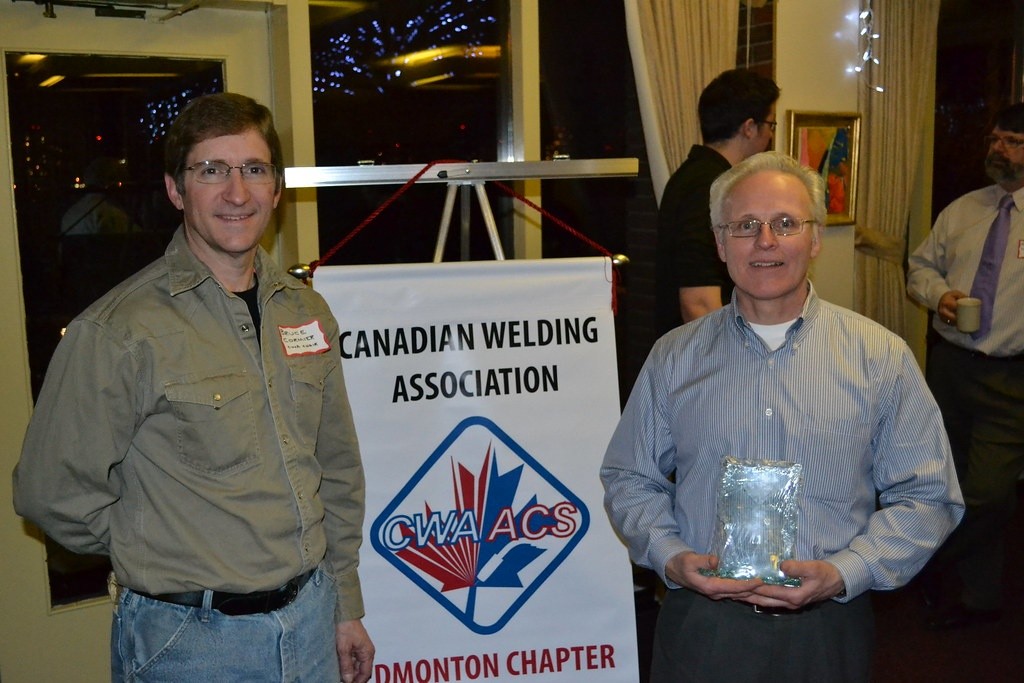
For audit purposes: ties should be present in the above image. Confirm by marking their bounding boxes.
[968,197,1015,342]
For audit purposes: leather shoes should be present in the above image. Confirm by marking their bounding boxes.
[921,603,1006,628]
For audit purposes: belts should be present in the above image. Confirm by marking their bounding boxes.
[724,598,833,616]
[128,569,317,616]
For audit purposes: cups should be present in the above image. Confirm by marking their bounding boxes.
[958,293,982,336]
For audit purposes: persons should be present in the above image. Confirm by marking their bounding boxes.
[601,148,969,683]
[651,65,779,368]
[61,153,136,235]
[907,103,1023,623]
[11,91,376,683]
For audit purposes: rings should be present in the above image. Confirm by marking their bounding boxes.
[947,319,950,324]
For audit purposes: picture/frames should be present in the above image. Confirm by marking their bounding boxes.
[784,108,863,226]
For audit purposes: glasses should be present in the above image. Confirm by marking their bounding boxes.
[185,161,280,184]
[718,217,818,238]
[757,119,777,132]
[985,136,1024,149]
